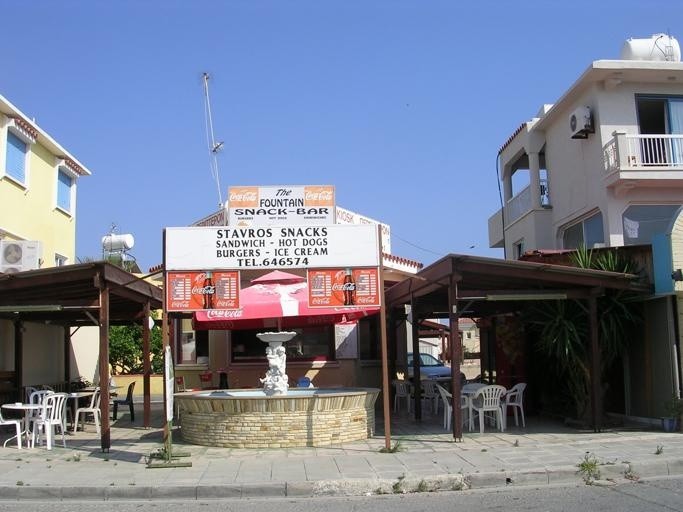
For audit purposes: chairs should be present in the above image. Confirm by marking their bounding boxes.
[0,376,136,450]
[393,378,528,435]
[200,373,217,390]
[175,376,198,431]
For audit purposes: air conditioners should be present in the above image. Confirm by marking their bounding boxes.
[1,239,43,266]
[0,266,40,274]
[568,106,593,139]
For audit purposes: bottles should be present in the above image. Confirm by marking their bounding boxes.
[218,372,229,390]
[202,271,214,310]
[342,267,356,306]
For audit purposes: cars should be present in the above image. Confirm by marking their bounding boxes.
[406,352,465,389]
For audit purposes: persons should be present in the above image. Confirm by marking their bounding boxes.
[258,345,291,397]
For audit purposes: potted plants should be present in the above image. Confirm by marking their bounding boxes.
[659,393,682,433]
